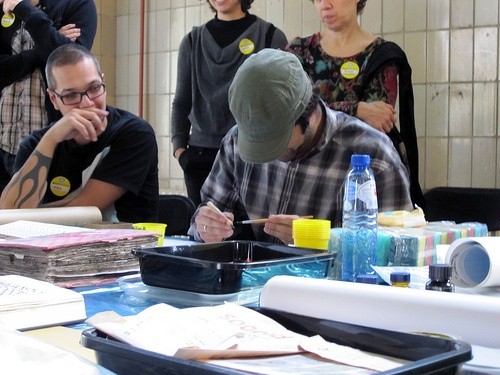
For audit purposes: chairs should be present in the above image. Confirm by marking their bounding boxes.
[144,194,195,237]
[220,199,257,243]
[413,186,500,237]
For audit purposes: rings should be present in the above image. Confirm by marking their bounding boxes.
[204,225,207,232]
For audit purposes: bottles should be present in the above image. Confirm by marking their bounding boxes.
[425,264,455,292]
[356,275,379,285]
[390,272,411,288]
[340,154,377,282]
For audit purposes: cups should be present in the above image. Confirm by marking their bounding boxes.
[292,219,331,251]
[132,222,167,247]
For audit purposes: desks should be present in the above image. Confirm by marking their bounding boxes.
[0,222,500,375]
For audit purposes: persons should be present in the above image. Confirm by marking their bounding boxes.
[0,0,97,194]
[284,0,423,210]
[0,43,160,220]
[187,50,414,246]
[170,0,289,240]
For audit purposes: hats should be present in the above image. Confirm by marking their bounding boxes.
[228,48,310,164]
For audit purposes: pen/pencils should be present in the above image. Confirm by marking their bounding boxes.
[235,215,314,224]
[207,201,235,229]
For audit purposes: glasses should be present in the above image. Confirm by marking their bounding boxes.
[50,78,106,105]
